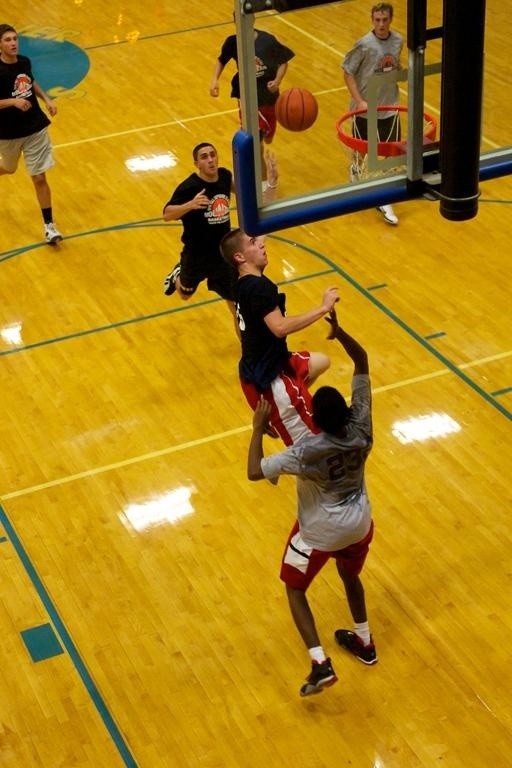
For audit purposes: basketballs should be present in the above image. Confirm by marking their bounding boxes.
[274,88,318,132]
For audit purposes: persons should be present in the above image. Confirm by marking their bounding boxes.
[340,3,404,225]
[210,8,296,195]
[219,147,342,445]
[0,23,63,245]
[163,143,242,342]
[246,305,379,698]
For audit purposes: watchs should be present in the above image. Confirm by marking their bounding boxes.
[266,183,279,191]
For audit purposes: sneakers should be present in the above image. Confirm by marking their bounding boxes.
[300,657,338,697]
[335,629,378,665]
[377,205,399,225]
[164,262,181,295]
[264,424,279,439]
[351,165,358,183]
[44,223,63,243]
